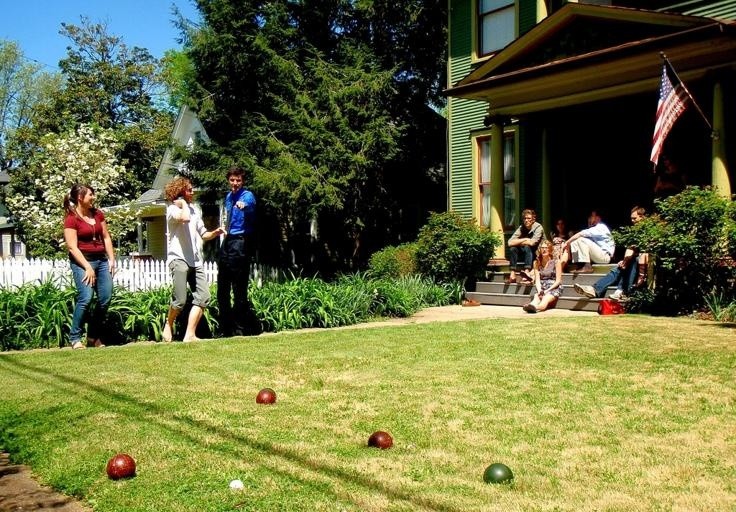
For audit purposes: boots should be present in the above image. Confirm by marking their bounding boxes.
[632,264,648,287]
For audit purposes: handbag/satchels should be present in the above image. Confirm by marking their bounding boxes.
[598,300,624,315]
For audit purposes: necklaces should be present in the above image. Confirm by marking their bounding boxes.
[77,213,96,241]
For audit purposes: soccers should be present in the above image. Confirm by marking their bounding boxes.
[368,430,394,448]
[484,465,514,484]
[257,388,277,404]
[108,453,134,479]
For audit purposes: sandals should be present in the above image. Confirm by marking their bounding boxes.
[463,298,480,306]
[71,339,84,349]
[93,337,105,347]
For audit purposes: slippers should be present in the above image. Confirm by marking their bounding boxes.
[520,271,533,281]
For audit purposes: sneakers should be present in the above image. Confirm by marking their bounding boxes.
[574,283,596,298]
[608,289,623,300]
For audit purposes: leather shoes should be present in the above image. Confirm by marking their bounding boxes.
[504,278,516,283]
[569,267,594,273]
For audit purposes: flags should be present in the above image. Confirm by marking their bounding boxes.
[648,60,696,173]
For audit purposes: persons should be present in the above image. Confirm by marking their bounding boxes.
[60,183,117,350]
[159,176,227,343]
[571,205,646,299]
[617,245,651,293]
[520,215,575,282]
[502,209,548,286]
[522,239,564,314]
[560,208,617,273]
[655,149,695,201]
[212,166,265,337]
